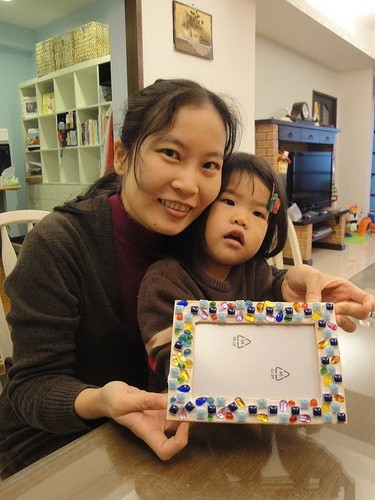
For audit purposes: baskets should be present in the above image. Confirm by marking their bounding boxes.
[34,20,110,78]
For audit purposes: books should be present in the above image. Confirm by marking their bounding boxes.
[82,118,100,146]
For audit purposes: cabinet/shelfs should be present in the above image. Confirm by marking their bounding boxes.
[255,118,350,266]
[18,56,114,210]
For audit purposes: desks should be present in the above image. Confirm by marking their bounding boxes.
[0,184,22,212]
[0,387,374,500]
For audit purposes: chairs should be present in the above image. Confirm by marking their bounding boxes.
[0,210,51,277]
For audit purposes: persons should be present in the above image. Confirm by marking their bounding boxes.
[136,152,289,389]
[0,79,375,482]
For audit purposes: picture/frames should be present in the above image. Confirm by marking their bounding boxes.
[166,300,348,425]
